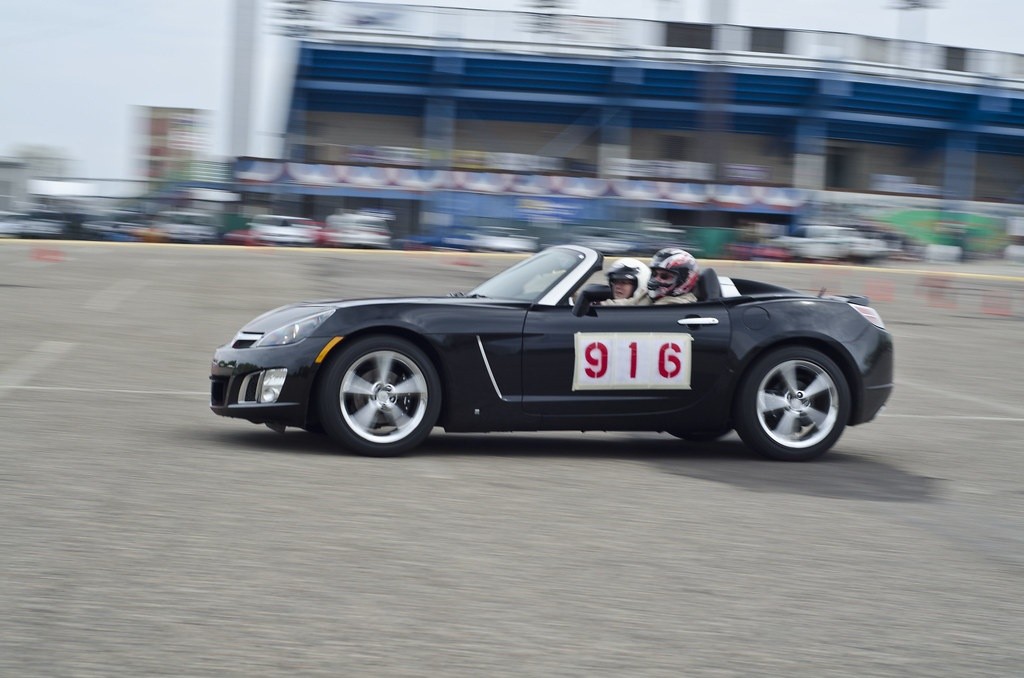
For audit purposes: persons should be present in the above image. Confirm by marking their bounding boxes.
[603,258,652,306]
[590,248,700,305]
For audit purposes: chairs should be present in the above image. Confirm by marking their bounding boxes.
[693,267,720,302]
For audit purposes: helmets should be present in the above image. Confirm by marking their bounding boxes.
[605,258,652,304]
[646,247,700,300]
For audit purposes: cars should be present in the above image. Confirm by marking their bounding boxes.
[0,211,28,237]
[20,209,65,240]
[248,215,318,246]
[443,226,539,253]
[149,210,218,245]
[776,226,890,263]
[324,214,391,249]
[210,243,896,462]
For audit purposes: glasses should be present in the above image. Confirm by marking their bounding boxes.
[652,271,674,280]
[610,278,637,286]
